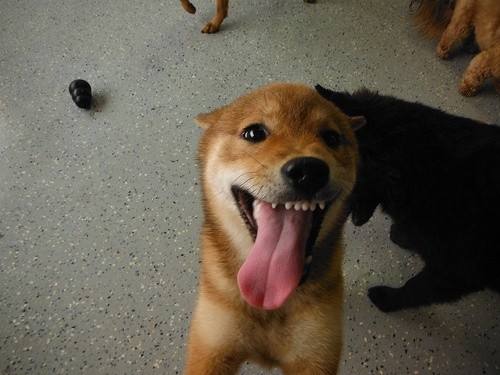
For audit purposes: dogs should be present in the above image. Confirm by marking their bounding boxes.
[185,81,367,375]
[311,88,500,313]
[408,0,500,95]
